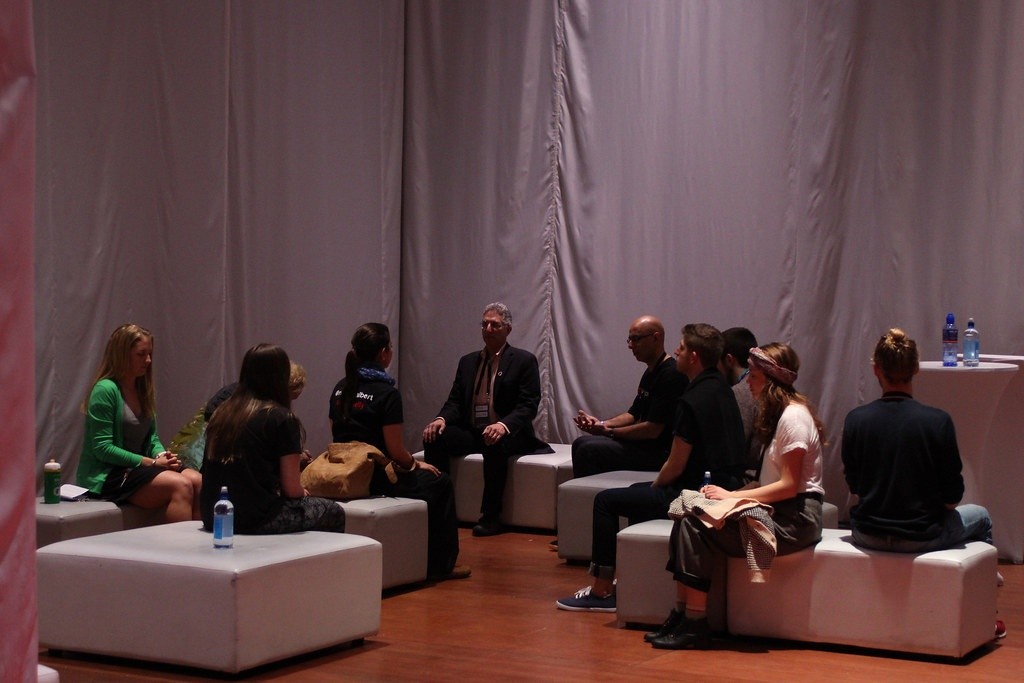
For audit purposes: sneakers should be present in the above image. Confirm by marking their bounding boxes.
[548,541,558,550]
[556,586,617,612]
[644,611,687,642]
[652,617,713,650]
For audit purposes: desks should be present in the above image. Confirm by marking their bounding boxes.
[911,354,1024,506]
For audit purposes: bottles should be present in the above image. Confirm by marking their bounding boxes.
[44,459,61,504]
[942,312,959,366]
[698,471,712,494]
[213,486,234,549]
[963,318,980,367]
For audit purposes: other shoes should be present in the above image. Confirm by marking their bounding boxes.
[473,518,504,537]
[453,564,472,578]
[995,621,1006,639]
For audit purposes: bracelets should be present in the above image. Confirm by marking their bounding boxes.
[601,421,604,424]
[152,461,156,468]
[156,451,166,458]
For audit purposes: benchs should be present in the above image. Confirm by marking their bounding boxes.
[32,444,998,674]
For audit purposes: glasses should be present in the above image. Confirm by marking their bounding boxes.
[628,334,652,343]
[480,322,507,329]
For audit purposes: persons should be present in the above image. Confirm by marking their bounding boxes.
[644,342,826,648]
[720,327,767,479]
[199,344,346,535]
[548,316,689,549]
[424,302,555,536]
[77,325,203,523]
[557,323,748,611]
[841,330,1006,637]
[203,362,313,468]
[329,322,471,580]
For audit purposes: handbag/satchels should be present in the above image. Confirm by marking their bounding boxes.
[299,441,402,498]
[165,406,211,472]
